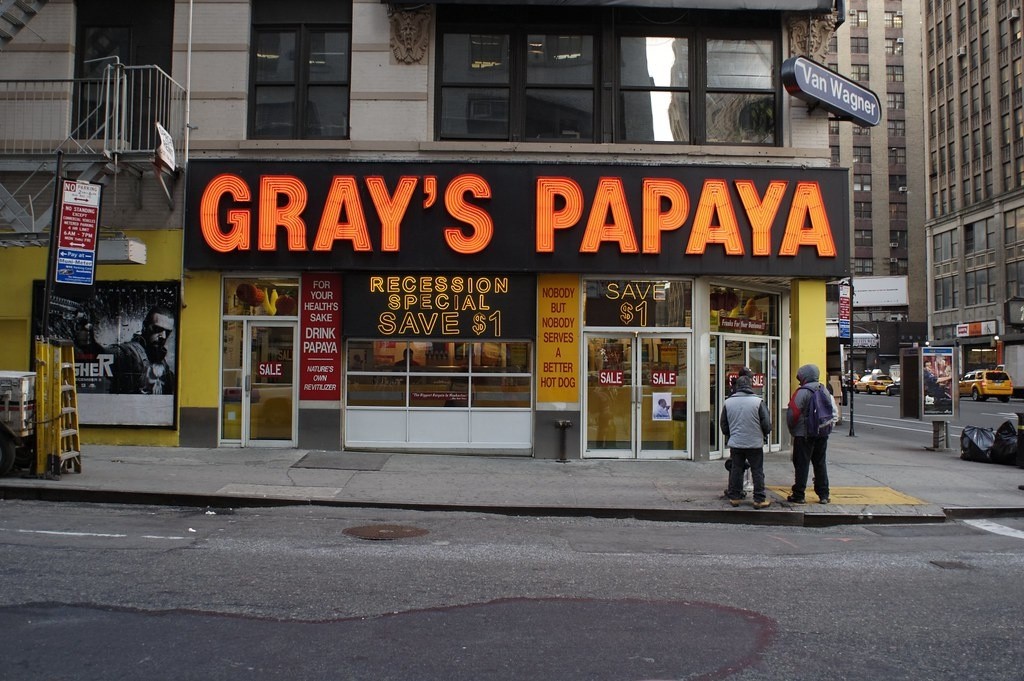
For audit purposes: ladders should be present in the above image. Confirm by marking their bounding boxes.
[31,334,83,481]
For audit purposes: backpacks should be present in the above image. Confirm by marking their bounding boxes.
[797,383,833,437]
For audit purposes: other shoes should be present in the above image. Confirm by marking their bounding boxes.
[729,498,742,507]
[819,498,831,504]
[787,495,805,504]
[753,498,771,509]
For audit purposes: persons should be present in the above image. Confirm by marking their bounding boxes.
[725,367,757,498]
[655,399,670,418]
[795,20,826,53]
[71,308,175,396]
[923,360,952,412]
[787,365,834,504]
[392,349,421,383]
[721,376,771,509]
[393,13,427,49]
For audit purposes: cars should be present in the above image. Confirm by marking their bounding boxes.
[587,368,686,441]
[883,375,900,396]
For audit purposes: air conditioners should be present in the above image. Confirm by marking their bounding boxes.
[1006,10,1019,21]
[892,243,898,247]
[896,11,903,15]
[898,187,907,191]
[956,47,965,57]
[897,38,904,43]
[850,9,856,14]
[890,258,897,262]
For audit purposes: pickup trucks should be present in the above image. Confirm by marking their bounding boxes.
[224,371,403,446]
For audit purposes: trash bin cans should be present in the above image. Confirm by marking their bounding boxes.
[1015,411,1024,469]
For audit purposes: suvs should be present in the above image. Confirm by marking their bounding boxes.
[415,364,531,408]
[854,373,894,394]
[844,373,860,393]
[959,369,1013,402]
